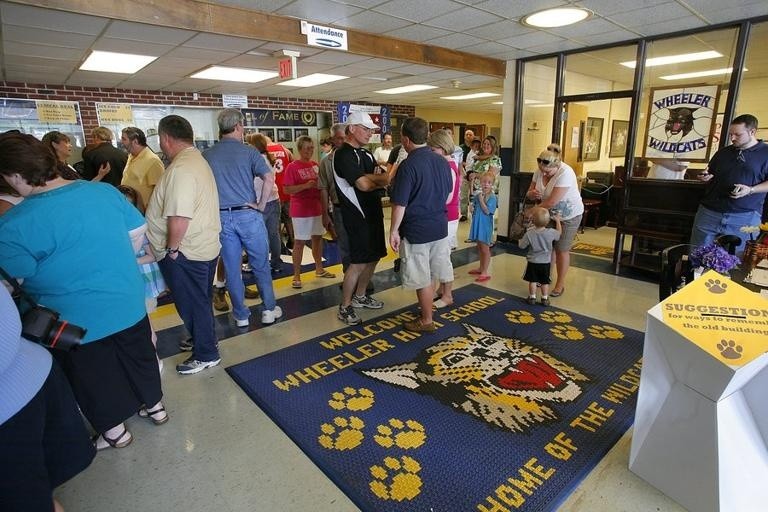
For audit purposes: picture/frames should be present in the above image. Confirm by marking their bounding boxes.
[258,128,273,141]
[583,116,604,162]
[608,119,629,158]
[294,128,308,141]
[276,128,292,141]
[243,127,255,142]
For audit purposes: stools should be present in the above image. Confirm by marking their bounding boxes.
[578,198,601,233]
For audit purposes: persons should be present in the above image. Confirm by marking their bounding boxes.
[0,281,103,511]
[688,113,768,265]
[0,127,170,452]
[643,159,690,180]
[524,142,586,300]
[516,204,562,308]
[283,112,505,329]
[2,107,282,377]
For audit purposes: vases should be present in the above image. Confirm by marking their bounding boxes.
[742,240,767,274]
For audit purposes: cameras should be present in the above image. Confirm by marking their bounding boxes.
[21,305,86,352]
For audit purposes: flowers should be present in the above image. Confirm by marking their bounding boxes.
[739,222,767,244]
[688,241,741,276]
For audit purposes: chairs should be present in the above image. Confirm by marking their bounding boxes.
[712,232,741,256]
[658,242,703,303]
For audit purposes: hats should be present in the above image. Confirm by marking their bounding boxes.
[346,111,381,131]
[472,136,481,143]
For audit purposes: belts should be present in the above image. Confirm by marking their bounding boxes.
[221,206,250,211]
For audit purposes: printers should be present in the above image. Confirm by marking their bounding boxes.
[586,170,614,186]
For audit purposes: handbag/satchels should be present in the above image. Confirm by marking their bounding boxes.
[509,197,539,240]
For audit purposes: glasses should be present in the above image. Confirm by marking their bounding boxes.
[537,158,550,164]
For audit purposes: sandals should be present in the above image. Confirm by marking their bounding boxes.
[139,406,169,424]
[90,428,132,450]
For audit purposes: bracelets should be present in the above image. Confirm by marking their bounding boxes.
[749,184,755,196]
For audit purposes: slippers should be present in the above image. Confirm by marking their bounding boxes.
[315,270,336,278]
[475,275,490,282]
[404,292,454,332]
[468,269,481,274]
[292,279,301,288]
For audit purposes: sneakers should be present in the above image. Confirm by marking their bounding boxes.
[337,281,383,326]
[460,216,467,221]
[464,239,473,243]
[177,338,221,375]
[528,288,564,306]
[213,262,282,326]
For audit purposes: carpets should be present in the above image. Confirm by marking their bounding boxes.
[222,280,645,511]
